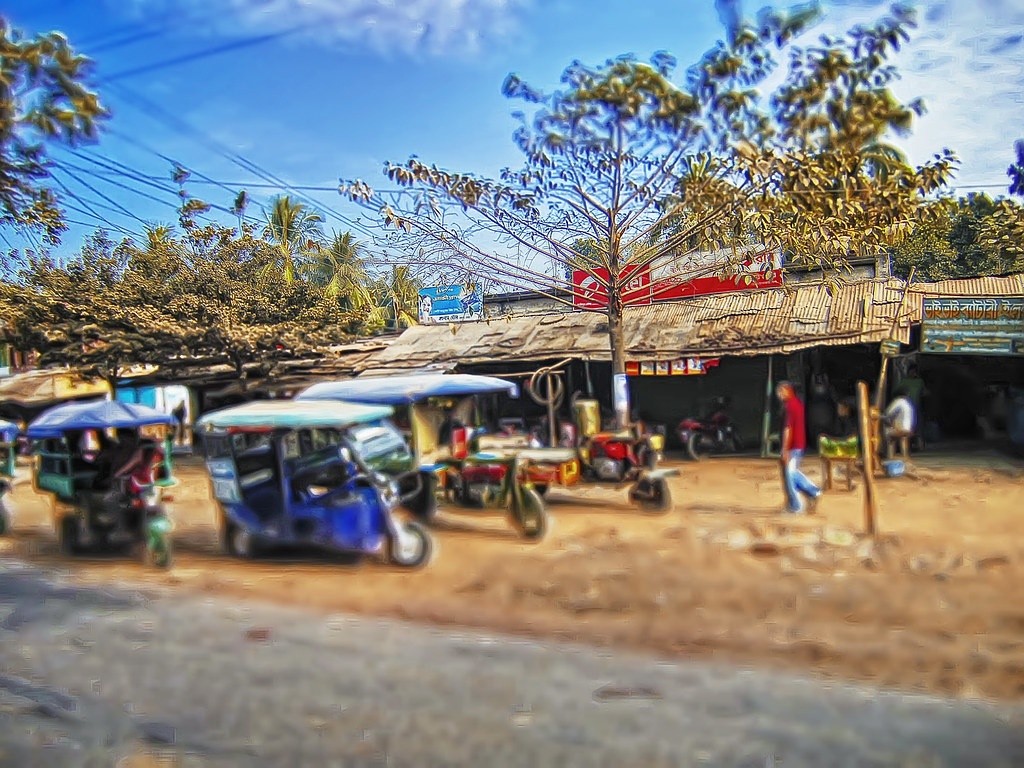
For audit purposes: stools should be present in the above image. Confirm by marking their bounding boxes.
[897,433,914,460]
[820,455,856,492]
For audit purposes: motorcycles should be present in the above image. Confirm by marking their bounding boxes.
[28,396,182,572]
[198,400,435,569]
[298,375,552,541]
[0,421,19,532]
[680,396,743,462]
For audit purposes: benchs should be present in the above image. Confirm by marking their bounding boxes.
[209,444,283,507]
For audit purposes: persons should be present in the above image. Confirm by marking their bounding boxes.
[879,388,913,457]
[95,429,167,502]
[776,381,820,513]
[171,400,186,446]
[421,295,434,321]
[438,407,463,503]
